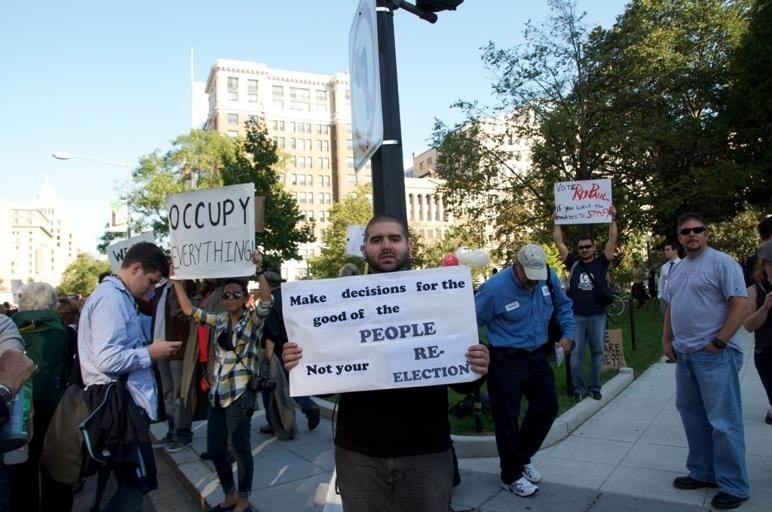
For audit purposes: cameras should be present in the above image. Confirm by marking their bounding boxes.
[249,376,276,394]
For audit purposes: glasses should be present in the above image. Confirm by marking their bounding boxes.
[221,292,241,299]
[578,245,591,249]
[680,227,705,235]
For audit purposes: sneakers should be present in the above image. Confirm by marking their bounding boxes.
[590,391,601,400]
[501,464,542,496]
[151,437,191,452]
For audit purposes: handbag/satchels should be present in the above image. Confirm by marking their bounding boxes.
[594,279,614,306]
[40,384,89,486]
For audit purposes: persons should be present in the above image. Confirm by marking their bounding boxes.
[1,268,323,511]
[76,238,185,512]
[744,214,772,287]
[167,251,275,511]
[549,205,622,403]
[280,213,493,512]
[742,235,772,425]
[627,259,649,309]
[658,211,752,511]
[474,242,577,497]
[645,259,658,299]
[657,240,683,364]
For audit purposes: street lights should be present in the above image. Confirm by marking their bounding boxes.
[52,150,134,239]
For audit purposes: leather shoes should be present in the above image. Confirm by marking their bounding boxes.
[260,423,276,433]
[711,491,748,509]
[674,477,720,489]
[308,404,320,430]
[211,503,237,512]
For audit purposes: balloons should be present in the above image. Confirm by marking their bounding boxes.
[443,244,488,272]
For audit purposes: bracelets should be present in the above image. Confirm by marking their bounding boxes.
[710,336,727,350]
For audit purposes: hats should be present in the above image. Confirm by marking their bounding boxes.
[264,271,285,282]
[516,244,548,281]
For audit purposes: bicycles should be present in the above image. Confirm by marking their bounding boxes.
[607,291,625,317]
